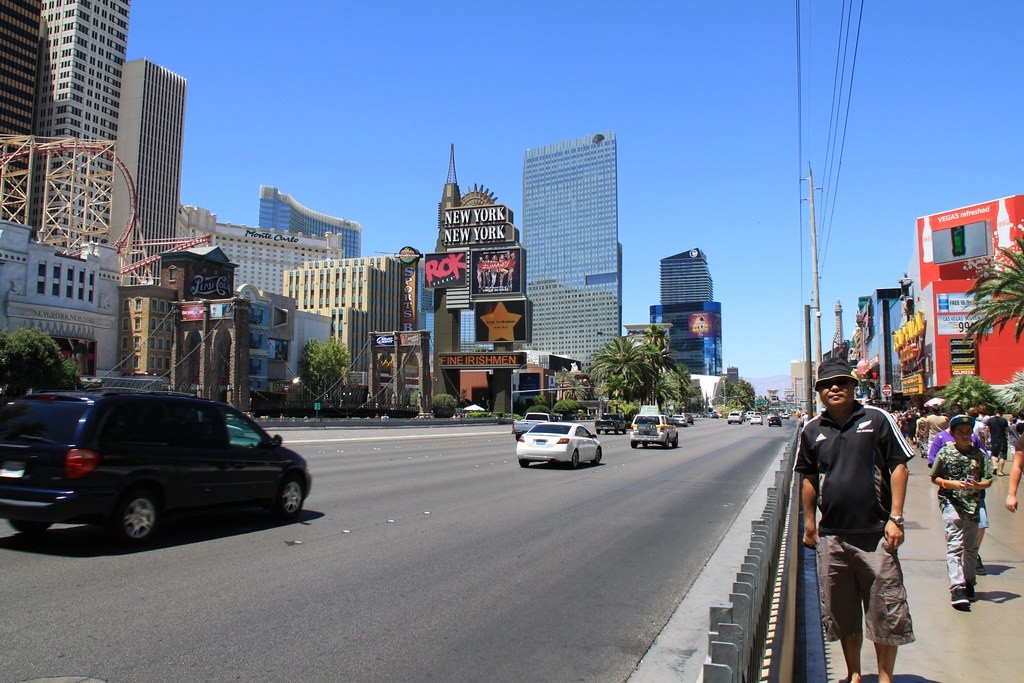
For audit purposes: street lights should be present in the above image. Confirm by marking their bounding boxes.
[511,363,540,421]
[597,331,624,418]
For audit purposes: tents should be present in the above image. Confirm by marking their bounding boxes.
[464,404,485,412]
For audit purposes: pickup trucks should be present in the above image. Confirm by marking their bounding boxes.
[511,412,554,440]
[595,414,627,434]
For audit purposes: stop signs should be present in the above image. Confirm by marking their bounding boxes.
[882,385,891,395]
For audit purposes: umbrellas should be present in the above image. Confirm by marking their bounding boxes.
[924,398,946,407]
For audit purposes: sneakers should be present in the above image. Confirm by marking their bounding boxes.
[965,582,975,602]
[992,468,997,474]
[949,582,968,607]
[975,554,986,575]
[998,472,1004,476]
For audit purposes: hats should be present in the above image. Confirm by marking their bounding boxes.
[814,357,859,391]
[949,413,976,428]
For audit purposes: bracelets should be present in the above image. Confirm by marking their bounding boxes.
[942,480,948,489]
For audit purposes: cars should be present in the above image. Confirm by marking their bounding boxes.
[661,412,723,427]
[766,413,790,427]
[727,410,762,425]
[516,422,603,470]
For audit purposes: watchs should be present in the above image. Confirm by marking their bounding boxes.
[889,515,904,525]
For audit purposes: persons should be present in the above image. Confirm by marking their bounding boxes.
[793,358,917,683]
[375,413,389,420]
[930,414,995,605]
[477,253,515,288]
[885,407,1024,575]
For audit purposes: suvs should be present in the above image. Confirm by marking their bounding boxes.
[0,387,311,553]
[630,415,678,449]
